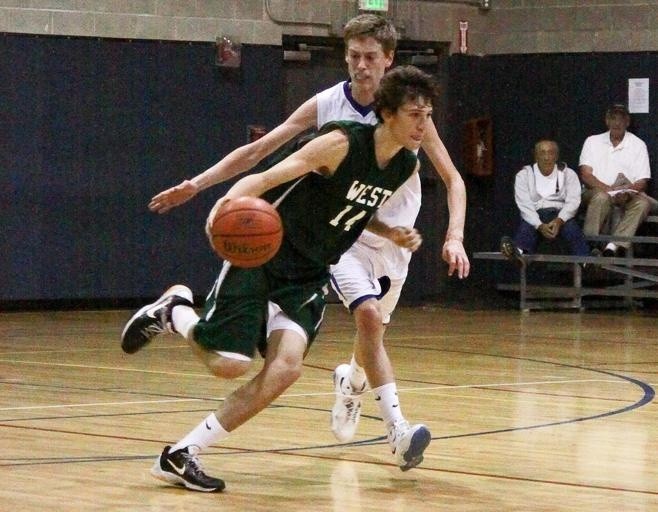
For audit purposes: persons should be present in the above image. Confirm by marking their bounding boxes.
[500,140,603,283]
[578,102,652,258]
[144,12,471,471]
[121,67,440,491]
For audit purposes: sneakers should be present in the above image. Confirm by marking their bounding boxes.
[499,236,530,270]
[391,421,431,471]
[120,285,195,354]
[149,445,225,492]
[581,241,616,272]
[328,363,368,442]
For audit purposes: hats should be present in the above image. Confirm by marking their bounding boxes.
[607,102,629,116]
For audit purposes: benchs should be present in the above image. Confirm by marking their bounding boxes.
[472,213,657,311]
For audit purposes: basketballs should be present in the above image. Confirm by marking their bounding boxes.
[209,196,284,268]
